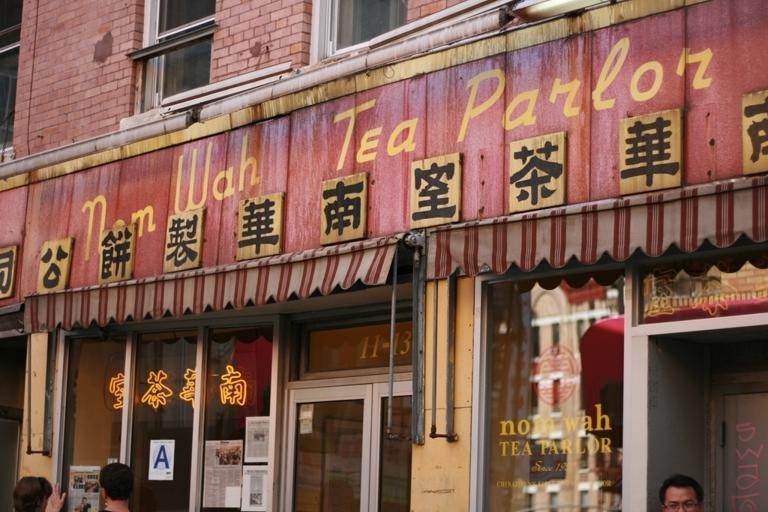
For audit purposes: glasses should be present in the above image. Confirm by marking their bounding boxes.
[662,502,698,509]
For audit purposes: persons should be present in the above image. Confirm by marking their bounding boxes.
[659,473,704,512]
[99,462,134,510]
[74,496,91,510]
[12,476,67,512]
